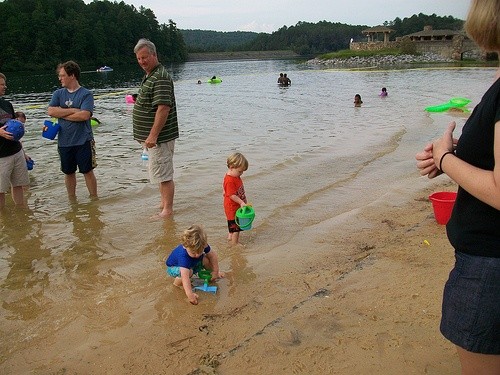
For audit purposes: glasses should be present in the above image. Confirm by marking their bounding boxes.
[57,74,69,80]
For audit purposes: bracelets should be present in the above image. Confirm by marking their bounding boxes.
[439,152,454,173]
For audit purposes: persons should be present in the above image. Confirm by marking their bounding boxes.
[278,73,291,86]
[0,74,32,207]
[166,225,224,305]
[353,94,363,104]
[198,75,217,84]
[380,87,388,96]
[223,153,252,244]
[48,61,98,200]
[133,39,179,219]
[416,0,500,375]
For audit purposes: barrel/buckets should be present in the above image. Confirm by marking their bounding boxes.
[428,192,457,224]
[235,206,255,230]
[42,117,60,140]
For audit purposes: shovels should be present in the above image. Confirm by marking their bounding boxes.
[193,269,218,294]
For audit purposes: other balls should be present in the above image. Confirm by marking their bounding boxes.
[4,120,25,141]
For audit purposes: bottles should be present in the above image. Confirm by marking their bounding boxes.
[141,148,149,167]
